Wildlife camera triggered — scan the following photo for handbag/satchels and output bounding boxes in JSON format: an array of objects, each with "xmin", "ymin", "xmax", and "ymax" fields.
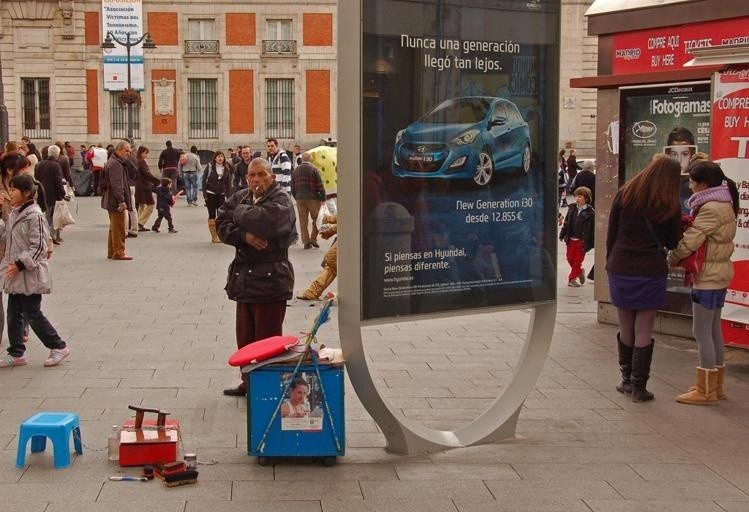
[
  {"xmin": 178, "ymin": 155, "xmax": 188, "ymax": 165},
  {"xmin": 52, "ymin": 201, "xmax": 76, "ymax": 231}
]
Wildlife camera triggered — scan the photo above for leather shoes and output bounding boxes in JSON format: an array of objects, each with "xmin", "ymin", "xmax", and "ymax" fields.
[
  {"xmin": 108, "ymin": 255, "xmax": 132, "ymax": 260},
  {"xmin": 297, "ymin": 296, "xmax": 310, "ymax": 300}
]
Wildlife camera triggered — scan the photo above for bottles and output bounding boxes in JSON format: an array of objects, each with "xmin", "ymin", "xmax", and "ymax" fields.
[
  {"xmin": 184, "ymin": 453, "xmax": 198, "ymax": 471},
  {"xmin": 107, "ymin": 425, "xmax": 121, "ymax": 461}
]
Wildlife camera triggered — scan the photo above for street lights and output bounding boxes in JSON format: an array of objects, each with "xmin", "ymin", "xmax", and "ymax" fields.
[{"xmin": 100, "ymin": 29, "xmax": 159, "ymax": 148}]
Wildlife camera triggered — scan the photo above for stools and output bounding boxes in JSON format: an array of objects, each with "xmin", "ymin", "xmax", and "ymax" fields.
[{"xmin": 15, "ymin": 412, "xmax": 83, "ymax": 468}]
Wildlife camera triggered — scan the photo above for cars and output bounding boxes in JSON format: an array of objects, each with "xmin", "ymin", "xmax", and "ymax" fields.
[
  {"xmin": 560, "ymin": 158, "xmax": 596, "ymax": 186},
  {"xmin": 389, "ymin": 93, "xmax": 531, "ymax": 190}
]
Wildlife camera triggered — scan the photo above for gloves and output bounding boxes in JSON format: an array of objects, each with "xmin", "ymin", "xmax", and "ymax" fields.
[
  {"xmin": 319, "ymin": 229, "xmax": 334, "ymax": 240},
  {"xmin": 63, "ymin": 194, "xmax": 70, "ymax": 201}
]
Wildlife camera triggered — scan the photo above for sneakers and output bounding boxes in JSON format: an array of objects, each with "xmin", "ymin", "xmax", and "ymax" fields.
[
  {"xmin": 304, "ymin": 242, "xmax": 319, "ymax": 249},
  {"xmin": 53, "ymin": 236, "xmax": 63, "ymax": 245},
  {"xmin": 187, "ymin": 200, "xmax": 198, "ymax": 206},
  {"xmin": 127, "ymin": 224, "xmax": 177, "ymax": 238},
  {"xmin": 44, "ymin": 347, "xmax": 70, "ymax": 366},
  {"xmin": 0, "ymin": 352, "xmax": 27, "ymax": 368}
]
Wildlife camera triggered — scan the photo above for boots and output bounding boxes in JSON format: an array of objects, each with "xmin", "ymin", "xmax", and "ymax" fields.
[{"xmin": 208, "ymin": 218, "xmax": 221, "ymax": 243}]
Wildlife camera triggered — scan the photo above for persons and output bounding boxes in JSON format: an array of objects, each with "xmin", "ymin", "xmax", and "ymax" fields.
[
  {"xmin": 281, "ymin": 380, "xmax": 310, "ymax": 420},
  {"xmin": 566, "ymin": 149, "xmax": 583, "ymax": 195},
  {"xmin": 568, "ymin": 159, "xmax": 596, "ymax": 210},
  {"xmin": 1, "ymin": 152, "xmax": 70, "ymax": 367},
  {"xmin": 604, "ymin": 152, "xmax": 684, "ymax": 403},
  {"xmin": 559, "ymin": 186, "xmax": 596, "ymax": 288},
  {"xmin": 0, "ymin": 136, "xmax": 337, "ymax": 259},
  {"xmin": 303, "ymin": 241, "xmax": 337, "ymax": 300},
  {"xmin": 560, "ymin": 150, "xmax": 568, "ymax": 183},
  {"xmin": 666, "ymin": 161, "xmax": 739, "ymax": 407},
  {"xmin": 215, "ymin": 157, "xmax": 297, "ymax": 396},
  {"xmin": 667, "ymin": 127, "xmax": 708, "ymax": 213}
]
